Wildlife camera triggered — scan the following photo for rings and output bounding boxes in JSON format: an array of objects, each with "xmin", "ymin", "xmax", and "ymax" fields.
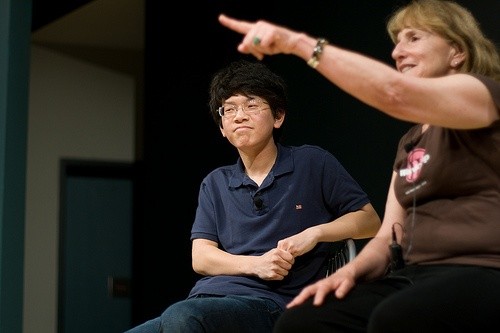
[{"xmin": 253, "ymin": 38, "xmax": 261, "ymax": 45}]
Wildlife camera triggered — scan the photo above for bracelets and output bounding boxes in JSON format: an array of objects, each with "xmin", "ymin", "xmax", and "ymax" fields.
[{"xmin": 307, "ymin": 38, "xmax": 327, "ymax": 70}]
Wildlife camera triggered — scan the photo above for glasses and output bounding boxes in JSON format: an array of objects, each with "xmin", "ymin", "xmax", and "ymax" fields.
[{"xmin": 217, "ymin": 99, "xmax": 271, "ymax": 118}]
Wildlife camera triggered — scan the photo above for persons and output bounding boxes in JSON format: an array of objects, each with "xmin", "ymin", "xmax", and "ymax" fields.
[
  {"xmin": 219, "ymin": 0, "xmax": 500, "ymax": 333},
  {"xmin": 126, "ymin": 64, "xmax": 380, "ymax": 333}
]
